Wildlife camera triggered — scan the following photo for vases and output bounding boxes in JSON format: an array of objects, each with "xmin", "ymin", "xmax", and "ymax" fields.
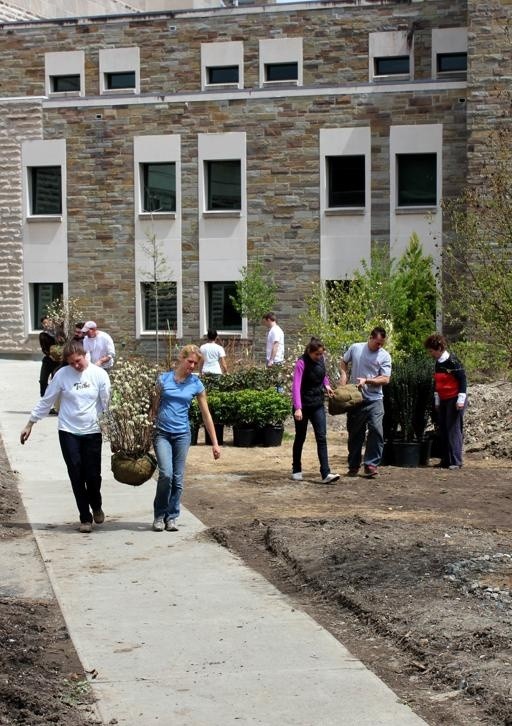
[
  {"xmin": 111, "ymin": 453, "xmax": 156, "ymax": 485},
  {"xmin": 329, "ymin": 384, "xmax": 362, "ymax": 414},
  {"xmin": 50, "ymin": 345, "xmax": 63, "ymax": 362}
]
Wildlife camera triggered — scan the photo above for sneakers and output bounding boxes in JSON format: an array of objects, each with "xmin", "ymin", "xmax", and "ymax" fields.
[
  {"xmin": 434, "ymin": 463, "xmax": 446, "ymax": 467},
  {"xmin": 348, "ymin": 467, "xmax": 358, "ymax": 476},
  {"xmin": 165, "ymin": 519, "xmax": 178, "ymax": 530},
  {"xmin": 322, "ymin": 474, "xmax": 340, "ymax": 483},
  {"xmin": 448, "ymin": 465, "xmax": 461, "ymax": 469},
  {"xmin": 154, "ymin": 514, "xmax": 165, "ymax": 531},
  {"xmin": 93, "ymin": 509, "xmax": 104, "ymax": 523},
  {"xmin": 80, "ymin": 523, "xmax": 92, "ymax": 531},
  {"xmin": 293, "ymin": 472, "xmax": 302, "ymax": 480},
  {"xmin": 364, "ymin": 465, "xmax": 379, "ymax": 476}
]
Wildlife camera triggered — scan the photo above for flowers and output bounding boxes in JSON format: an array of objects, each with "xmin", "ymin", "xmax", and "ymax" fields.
[
  {"xmin": 47, "ymin": 298, "xmax": 85, "ymax": 345},
  {"xmin": 97, "ymin": 355, "xmax": 157, "ymax": 456},
  {"xmin": 278, "ymin": 340, "xmax": 341, "ymax": 387}
]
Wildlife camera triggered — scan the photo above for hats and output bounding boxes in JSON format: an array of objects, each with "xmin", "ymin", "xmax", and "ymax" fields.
[{"xmin": 81, "ymin": 321, "xmax": 97, "ymax": 333}]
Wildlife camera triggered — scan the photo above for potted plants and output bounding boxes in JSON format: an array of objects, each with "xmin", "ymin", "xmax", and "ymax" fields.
[
  {"xmin": 188, "ymin": 396, "xmax": 199, "ymax": 445},
  {"xmin": 382, "ymin": 353, "xmax": 432, "ymax": 466},
  {"xmin": 199, "ymin": 391, "xmax": 226, "ymax": 446},
  {"xmin": 226, "ymin": 389, "xmax": 291, "ymax": 446}
]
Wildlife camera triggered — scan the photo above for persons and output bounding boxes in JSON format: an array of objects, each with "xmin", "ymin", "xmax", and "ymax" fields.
[
  {"xmin": 151, "ymin": 344, "xmax": 221, "ymax": 532},
  {"xmin": 48, "ymin": 322, "xmax": 85, "ymax": 415},
  {"xmin": 19, "ymin": 340, "xmax": 112, "ymax": 533},
  {"xmin": 80, "ymin": 320, "xmax": 115, "ymax": 372},
  {"xmin": 261, "ymin": 312, "xmax": 285, "ymax": 392},
  {"xmin": 198, "ymin": 327, "xmax": 228, "ymax": 378},
  {"xmin": 423, "ymin": 333, "xmax": 470, "ymax": 471},
  {"xmin": 39, "ymin": 316, "xmax": 66, "ymax": 397},
  {"xmin": 338, "ymin": 325, "xmax": 392, "ymax": 478},
  {"xmin": 290, "ymin": 335, "xmax": 341, "ymax": 484}
]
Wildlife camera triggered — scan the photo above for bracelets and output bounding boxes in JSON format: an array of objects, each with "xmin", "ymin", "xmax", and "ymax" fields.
[{"xmin": 365, "ymin": 377, "xmax": 367, "ymax": 384}]
